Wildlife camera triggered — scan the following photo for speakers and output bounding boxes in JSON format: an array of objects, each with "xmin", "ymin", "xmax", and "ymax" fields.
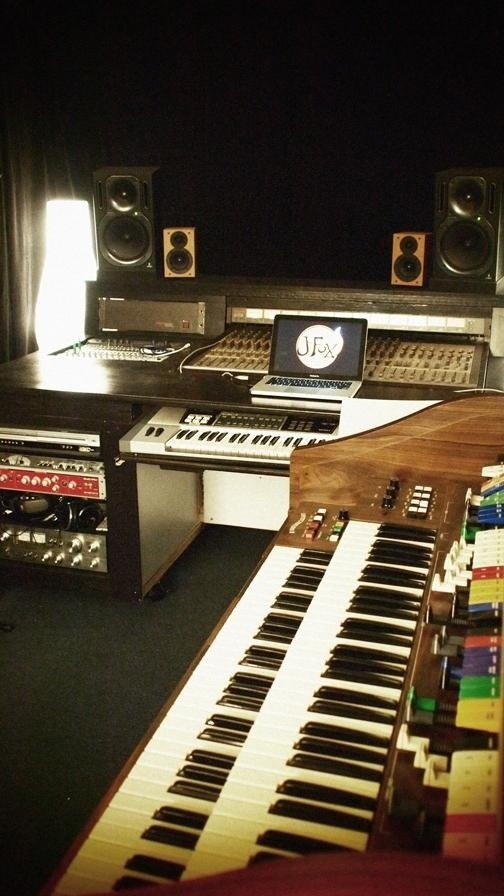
[
  {"xmin": 90, "ymin": 162, "xmax": 159, "ymax": 282},
  {"xmin": 390, "ymin": 231, "xmax": 433, "ymax": 287},
  {"xmin": 428, "ymin": 165, "xmax": 504, "ymax": 296},
  {"xmin": 162, "ymin": 226, "xmax": 196, "ymax": 279}
]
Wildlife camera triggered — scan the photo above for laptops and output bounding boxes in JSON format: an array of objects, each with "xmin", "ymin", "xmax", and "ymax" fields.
[{"xmin": 248, "ymin": 313, "xmax": 369, "ymax": 404}]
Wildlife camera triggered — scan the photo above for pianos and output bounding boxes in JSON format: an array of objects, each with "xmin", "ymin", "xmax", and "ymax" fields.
[
  {"xmin": 118, "ymin": 406, "xmax": 339, "ymax": 469},
  {"xmin": 53, "ymin": 393, "xmax": 502, "ymax": 896}
]
